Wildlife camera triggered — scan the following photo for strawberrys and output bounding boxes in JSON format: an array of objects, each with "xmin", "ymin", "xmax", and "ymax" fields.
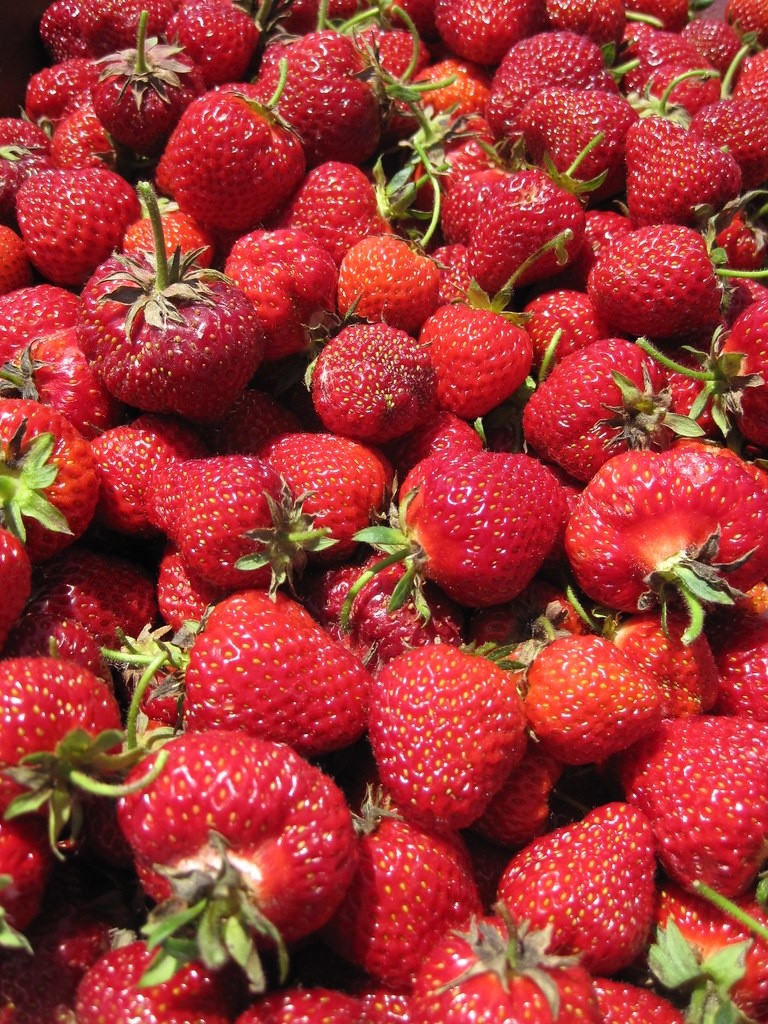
[{"xmin": 0, "ymin": 0, "xmax": 768, "ymax": 1024}]
